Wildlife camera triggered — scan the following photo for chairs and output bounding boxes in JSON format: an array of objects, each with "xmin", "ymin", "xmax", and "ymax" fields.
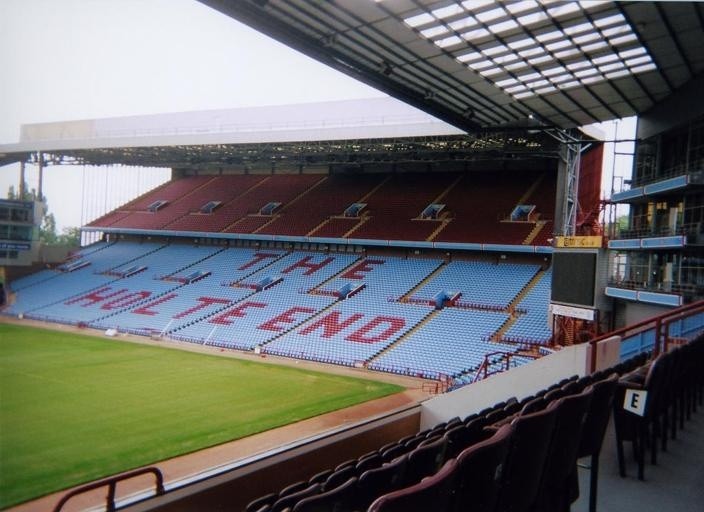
[
  {"xmin": 232, "ymin": 245, "xmax": 553, "ymax": 350},
  {"xmin": 83, "ymin": 175, "xmax": 557, "ymax": 247},
  {"xmin": 1, "ymin": 239, "xmax": 232, "ymax": 350},
  {"xmin": 242, "ymin": 336, "xmax": 703, "ymax": 512}
]
